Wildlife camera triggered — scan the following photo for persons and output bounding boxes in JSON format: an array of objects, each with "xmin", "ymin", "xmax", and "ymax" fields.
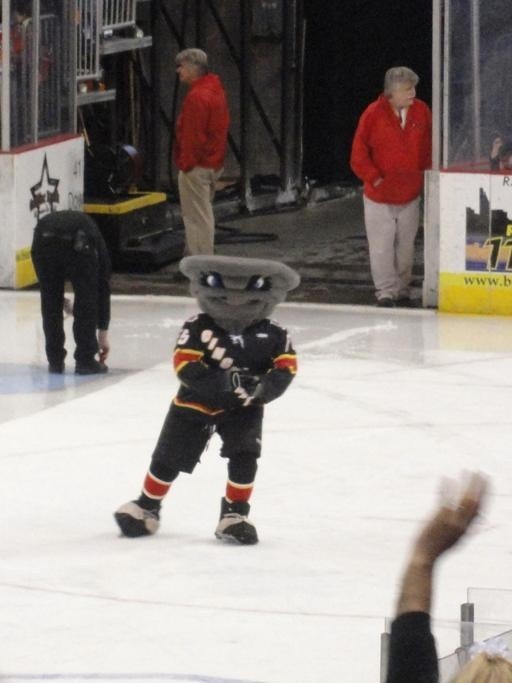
[
  {"xmin": 1, "ymin": 0, "xmax": 44, "ymax": 145},
  {"xmin": 28, "ymin": 210, "xmax": 115, "ymax": 372},
  {"xmin": 384, "ymin": 467, "xmax": 511, "ymax": 681},
  {"xmin": 174, "ymin": 47, "xmax": 233, "ymax": 258},
  {"xmin": 349, "ymin": 65, "xmax": 434, "ymax": 311},
  {"xmin": 488, "ymin": 137, "xmax": 512, "ymax": 170}
]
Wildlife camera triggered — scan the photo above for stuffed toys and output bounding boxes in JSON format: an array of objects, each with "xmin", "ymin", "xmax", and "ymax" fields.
[{"xmin": 116, "ymin": 253, "xmax": 302, "ymax": 546}]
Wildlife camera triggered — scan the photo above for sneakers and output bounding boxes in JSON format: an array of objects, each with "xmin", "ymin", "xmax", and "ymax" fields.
[
  {"xmin": 378, "ymin": 297, "xmax": 391, "ymax": 308},
  {"xmin": 47, "ymin": 363, "xmax": 66, "ymax": 373},
  {"xmin": 397, "ymin": 297, "xmax": 411, "ymax": 308},
  {"xmin": 76, "ymin": 361, "xmax": 109, "ymax": 374}
]
